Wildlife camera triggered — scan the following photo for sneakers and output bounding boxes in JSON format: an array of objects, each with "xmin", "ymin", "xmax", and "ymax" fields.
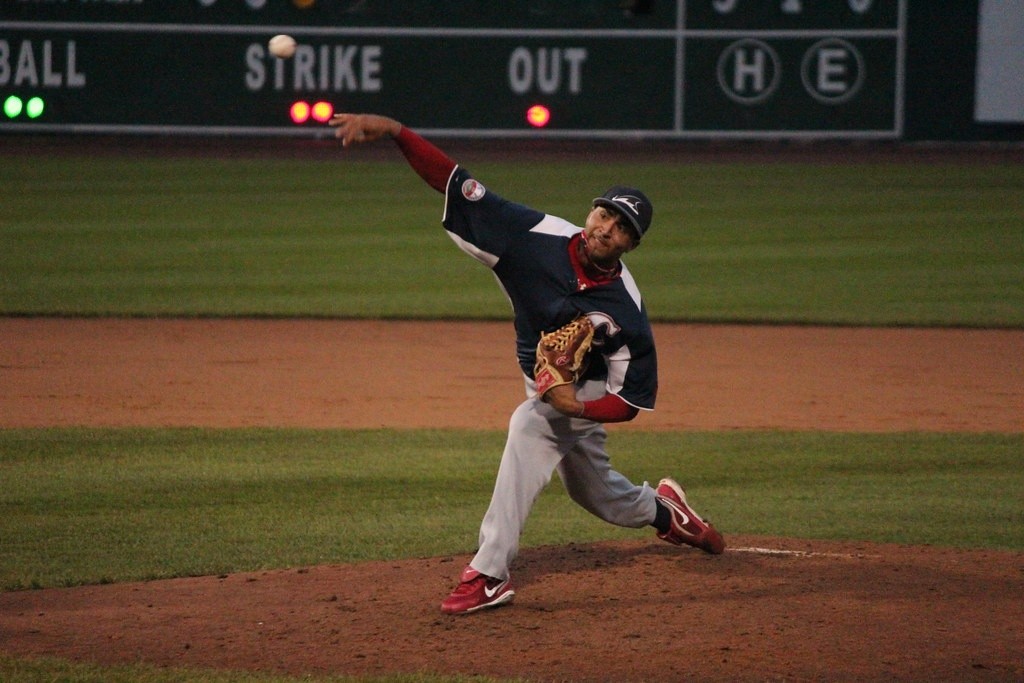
[
  {"xmin": 655, "ymin": 477, "xmax": 726, "ymax": 553},
  {"xmin": 442, "ymin": 567, "xmax": 516, "ymax": 615}
]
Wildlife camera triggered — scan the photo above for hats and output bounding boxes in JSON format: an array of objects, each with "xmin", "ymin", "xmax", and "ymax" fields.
[{"xmin": 593, "ymin": 185, "xmax": 654, "ymax": 238}]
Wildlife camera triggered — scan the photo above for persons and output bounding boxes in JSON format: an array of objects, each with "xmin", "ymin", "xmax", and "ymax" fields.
[{"xmin": 327, "ymin": 111, "xmax": 726, "ymax": 615}]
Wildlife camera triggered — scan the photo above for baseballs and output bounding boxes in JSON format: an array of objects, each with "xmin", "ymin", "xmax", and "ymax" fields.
[{"xmin": 268, "ymin": 34, "xmax": 296, "ymax": 58}]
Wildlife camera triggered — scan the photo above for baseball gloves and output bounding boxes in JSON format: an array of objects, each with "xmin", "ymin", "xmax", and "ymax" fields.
[{"xmin": 533, "ymin": 315, "xmax": 594, "ymax": 403}]
